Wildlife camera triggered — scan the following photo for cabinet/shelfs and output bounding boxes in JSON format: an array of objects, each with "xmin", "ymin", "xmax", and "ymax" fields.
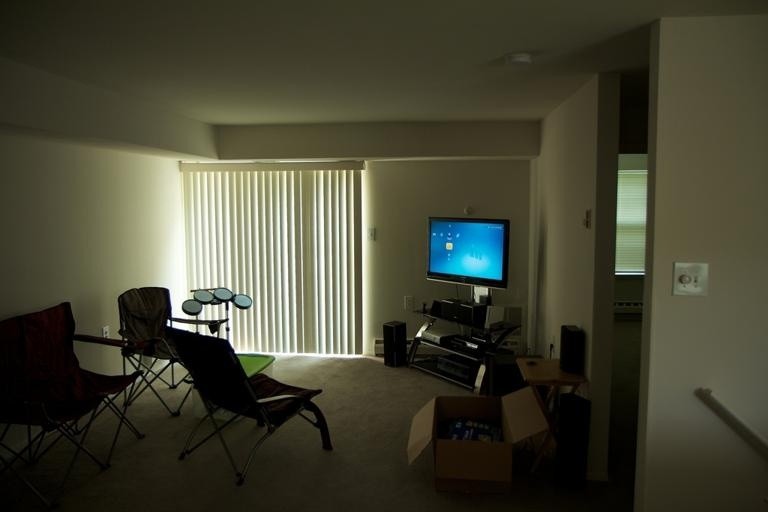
[{"xmin": 406, "ymin": 309, "xmax": 523, "ymax": 397}]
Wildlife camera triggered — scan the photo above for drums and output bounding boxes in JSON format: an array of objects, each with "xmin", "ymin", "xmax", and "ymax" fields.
[
  {"xmin": 214, "ymin": 289, "xmax": 233, "ymax": 300},
  {"xmin": 182, "ymin": 299, "xmax": 202, "ymax": 315},
  {"xmin": 233, "ymin": 294, "xmax": 252, "ymax": 309},
  {"xmin": 194, "ymin": 290, "xmax": 213, "ymax": 304}
]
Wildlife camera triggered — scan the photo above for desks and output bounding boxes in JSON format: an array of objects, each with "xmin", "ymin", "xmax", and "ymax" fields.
[{"xmin": 514, "ymin": 357, "xmax": 586, "ymax": 488}]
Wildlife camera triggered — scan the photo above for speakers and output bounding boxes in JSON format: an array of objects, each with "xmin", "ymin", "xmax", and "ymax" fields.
[
  {"xmin": 382, "ymin": 320, "xmax": 408, "ymax": 368},
  {"xmin": 559, "ymin": 324, "xmax": 586, "ymax": 375}
]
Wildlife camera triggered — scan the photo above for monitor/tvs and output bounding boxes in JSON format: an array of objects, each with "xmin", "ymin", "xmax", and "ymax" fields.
[{"xmin": 425, "ymin": 216, "xmax": 510, "ymax": 292}]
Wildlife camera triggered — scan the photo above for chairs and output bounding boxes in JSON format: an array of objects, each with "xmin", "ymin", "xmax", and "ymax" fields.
[
  {"xmin": 116, "ymin": 285, "xmax": 213, "ymax": 418},
  {"xmin": 1, "ymin": 300, "xmax": 145, "ymax": 509},
  {"xmin": 163, "ymin": 325, "xmax": 333, "ymax": 487}
]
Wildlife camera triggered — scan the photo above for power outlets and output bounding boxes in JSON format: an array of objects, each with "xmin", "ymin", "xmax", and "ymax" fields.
[{"xmin": 102, "ymin": 324, "xmax": 110, "ymax": 339}]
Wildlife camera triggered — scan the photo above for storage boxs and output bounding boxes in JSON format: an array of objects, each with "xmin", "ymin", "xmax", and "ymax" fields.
[
  {"xmin": 406, "ymin": 385, "xmax": 550, "ymax": 498},
  {"xmin": 184, "ymin": 353, "xmax": 275, "ymax": 424}
]
